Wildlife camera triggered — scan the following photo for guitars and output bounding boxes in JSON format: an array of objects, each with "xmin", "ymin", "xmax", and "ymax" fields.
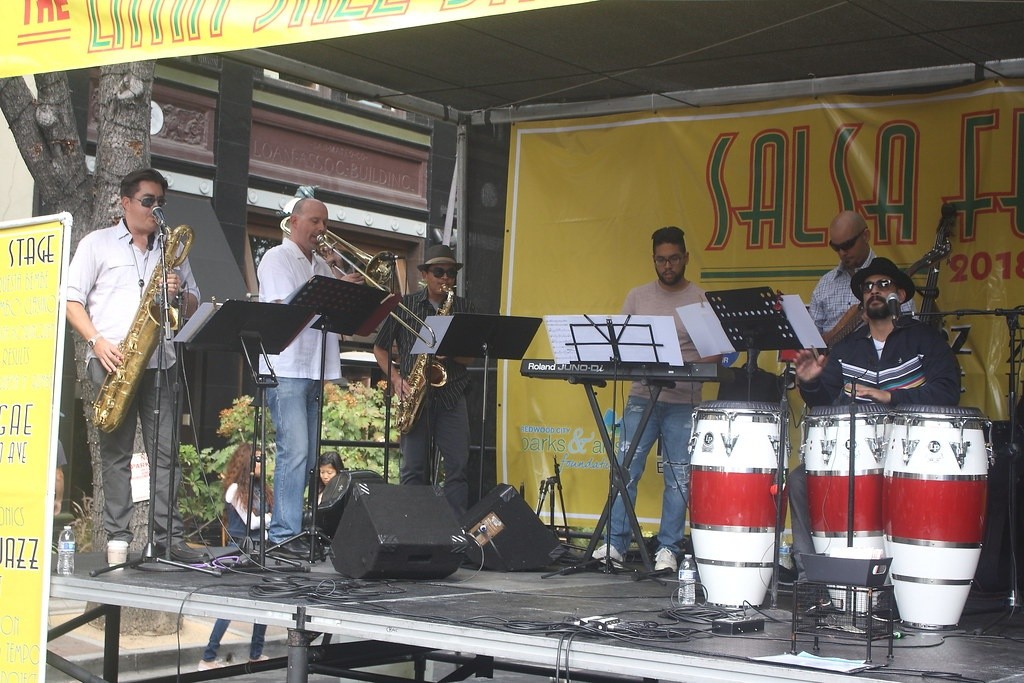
[
  {"xmin": 795, "ymin": 238, "xmax": 953, "ymax": 385},
  {"xmin": 916, "ymin": 201, "xmax": 959, "ymax": 325}
]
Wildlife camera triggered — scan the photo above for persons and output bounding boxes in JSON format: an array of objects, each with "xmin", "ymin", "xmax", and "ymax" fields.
[
  {"xmin": 197, "ymin": 442, "xmax": 274, "ymax": 671},
  {"xmin": 54, "ymin": 438, "xmax": 68, "ymax": 518},
  {"xmin": 257, "ymin": 197, "xmax": 379, "ymax": 556},
  {"xmin": 593, "ymin": 228, "xmax": 721, "ymax": 571},
  {"xmin": 307, "ymin": 450, "xmax": 344, "ymax": 510},
  {"xmin": 59, "ymin": 169, "xmax": 210, "ymax": 563},
  {"xmin": 805, "ymin": 210, "xmax": 919, "ymax": 406},
  {"xmin": 793, "ymin": 256, "xmax": 961, "ymax": 593},
  {"xmin": 374, "ymin": 245, "xmax": 474, "ymax": 526}
]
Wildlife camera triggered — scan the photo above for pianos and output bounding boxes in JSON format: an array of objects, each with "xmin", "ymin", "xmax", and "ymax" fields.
[{"xmin": 519, "ymin": 359, "xmax": 730, "ymax": 582}]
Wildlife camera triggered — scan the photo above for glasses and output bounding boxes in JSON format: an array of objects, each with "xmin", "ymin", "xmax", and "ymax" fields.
[
  {"xmin": 653, "ymin": 254, "xmax": 687, "ymax": 267},
  {"xmin": 828, "ymin": 227, "xmax": 868, "ymax": 253},
  {"xmin": 859, "ymin": 278, "xmax": 899, "ymax": 293},
  {"xmin": 129, "ymin": 195, "xmax": 167, "ymax": 208},
  {"xmin": 424, "ymin": 267, "xmax": 458, "ymax": 279}
]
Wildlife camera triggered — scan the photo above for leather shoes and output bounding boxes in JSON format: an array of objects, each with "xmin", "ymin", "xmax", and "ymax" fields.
[
  {"xmin": 156, "ymin": 541, "xmax": 210, "ymax": 564},
  {"xmin": 266, "ymin": 538, "xmax": 311, "ymax": 559},
  {"xmin": 105, "ymin": 544, "xmax": 130, "ymax": 563}
]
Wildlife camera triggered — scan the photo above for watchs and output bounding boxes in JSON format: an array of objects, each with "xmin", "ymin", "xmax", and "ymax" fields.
[
  {"xmin": 171, "ymin": 293, "xmax": 183, "ymax": 308},
  {"xmin": 87, "ymin": 334, "xmax": 100, "ymax": 348}
]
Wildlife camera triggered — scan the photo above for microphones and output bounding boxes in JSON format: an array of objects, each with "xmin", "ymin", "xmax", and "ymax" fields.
[
  {"xmin": 886, "ymin": 293, "xmax": 900, "ymax": 316},
  {"xmin": 380, "ymin": 252, "xmax": 404, "ymax": 259},
  {"xmin": 152, "ymin": 206, "xmax": 166, "ymax": 228}
]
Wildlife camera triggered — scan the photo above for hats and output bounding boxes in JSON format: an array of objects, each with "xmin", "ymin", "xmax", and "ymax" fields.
[
  {"xmin": 850, "ymin": 257, "xmax": 915, "ymax": 305},
  {"xmin": 417, "ymin": 245, "xmax": 464, "ymax": 272}
]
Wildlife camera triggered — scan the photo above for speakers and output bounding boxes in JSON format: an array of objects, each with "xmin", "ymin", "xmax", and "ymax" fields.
[
  {"xmin": 460, "ymin": 485, "xmax": 562, "ymax": 571},
  {"xmin": 327, "ymin": 483, "xmax": 467, "ymax": 579}
]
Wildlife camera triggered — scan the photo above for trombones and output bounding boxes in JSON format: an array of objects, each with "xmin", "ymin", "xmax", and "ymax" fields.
[{"xmin": 280, "ymin": 215, "xmax": 436, "ymax": 349}]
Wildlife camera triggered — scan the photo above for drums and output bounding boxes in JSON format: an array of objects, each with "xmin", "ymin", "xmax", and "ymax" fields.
[
  {"xmin": 801, "ymin": 404, "xmax": 891, "ymax": 617},
  {"xmin": 882, "ymin": 402, "xmax": 989, "ymax": 635},
  {"xmin": 688, "ymin": 401, "xmax": 790, "ymax": 609}
]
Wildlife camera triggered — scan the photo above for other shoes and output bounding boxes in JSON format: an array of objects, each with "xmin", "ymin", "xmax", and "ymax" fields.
[
  {"xmin": 258, "ymin": 654, "xmax": 269, "ymax": 661},
  {"xmin": 198, "ymin": 660, "xmax": 224, "ymax": 671}
]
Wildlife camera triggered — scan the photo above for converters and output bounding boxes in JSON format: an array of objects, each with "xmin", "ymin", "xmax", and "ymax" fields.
[{"xmin": 711, "ymin": 618, "xmax": 764, "ymax": 636}]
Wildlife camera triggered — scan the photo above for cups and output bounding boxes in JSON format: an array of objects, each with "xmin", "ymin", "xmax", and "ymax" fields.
[{"xmin": 108, "ymin": 540, "xmax": 129, "ymax": 571}]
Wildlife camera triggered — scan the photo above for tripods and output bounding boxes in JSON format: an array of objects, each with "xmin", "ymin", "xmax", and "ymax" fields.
[
  {"xmin": 539, "ymin": 314, "xmax": 666, "ymax": 589},
  {"xmin": 88, "ymin": 228, "xmax": 389, "ymax": 578},
  {"xmin": 894, "ymin": 309, "xmax": 1024, "ymax": 636}
]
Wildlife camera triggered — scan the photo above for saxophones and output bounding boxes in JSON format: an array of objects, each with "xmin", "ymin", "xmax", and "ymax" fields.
[
  {"xmin": 388, "ymin": 283, "xmax": 467, "ymax": 435},
  {"xmin": 90, "ymin": 206, "xmax": 202, "ymax": 434}
]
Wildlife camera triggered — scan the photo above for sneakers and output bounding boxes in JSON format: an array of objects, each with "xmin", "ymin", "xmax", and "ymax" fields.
[
  {"xmin": 592, "ymin": 543, "xmax": 624, "ymax": 565},
  {"xmin": 654, "ymin": 547, "xmax": 677, "ymax": 573}
]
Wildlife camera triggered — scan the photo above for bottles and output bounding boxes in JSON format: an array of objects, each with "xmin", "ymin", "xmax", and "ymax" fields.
[
  {"xmin": 678, "ymin": 554, "xmax": 697, "ymax": 605},
  {"xmin": 779, "ymin": 530, "xmax": 795, "ymax": 569},
  {"xmin": 56, "ymin": 525, "xmax": 75, "ymax": 575}
]
